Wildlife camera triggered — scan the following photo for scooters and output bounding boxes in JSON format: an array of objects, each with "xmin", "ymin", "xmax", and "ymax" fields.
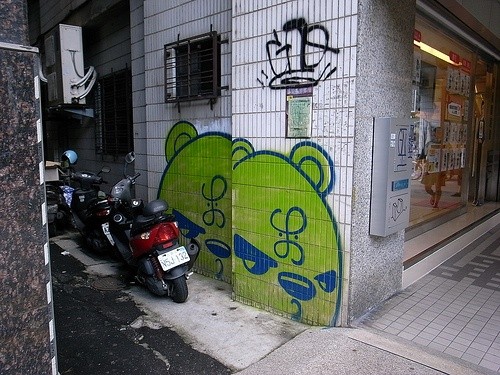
[
  {"xmin": 95, "ymin": 153, "xmax": 199, "ymax": 303},
  {"xmin": 40, "ymin": 152, "xmax": 81, "ymax": 237},
  {"xmin": 56, "ymin": 151, "xmax": 119, "ymax": 257}
]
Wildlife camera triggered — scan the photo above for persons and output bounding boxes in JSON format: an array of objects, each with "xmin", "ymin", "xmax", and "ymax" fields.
[{"xmin": 414, "ymin": 126, "xmax": 456, "ymax": 208}]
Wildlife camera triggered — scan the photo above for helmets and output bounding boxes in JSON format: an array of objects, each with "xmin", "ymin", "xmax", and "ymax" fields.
[{"xmin": 60, "ymin": 150, "xmax": 78, "ymax": 164}]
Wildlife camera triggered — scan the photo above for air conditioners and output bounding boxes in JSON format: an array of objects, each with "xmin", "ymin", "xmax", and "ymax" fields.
[{"xmin": 42, "ymin": 24, "xmax": 86, "ymax": 108}]
